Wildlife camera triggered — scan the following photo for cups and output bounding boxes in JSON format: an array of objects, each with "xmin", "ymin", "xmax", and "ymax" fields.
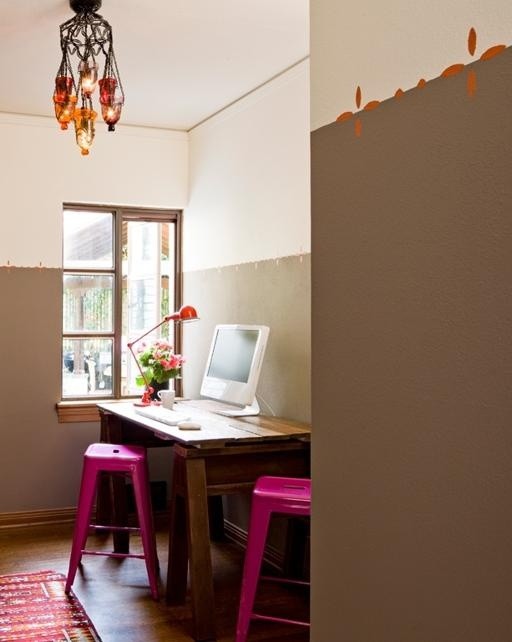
[{"xmin": 157, "ymin": 390, "xmax": 176, "ymax": 410}]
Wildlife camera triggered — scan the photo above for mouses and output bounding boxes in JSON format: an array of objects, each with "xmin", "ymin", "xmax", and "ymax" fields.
[{"xmin": 176, "ymin": 421, "xmax": 202, "ymax": 431}]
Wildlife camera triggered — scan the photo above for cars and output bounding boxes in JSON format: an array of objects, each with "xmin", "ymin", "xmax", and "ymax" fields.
[{"xmin": 64, "ymin": 351, "xmax": 90, "ymax": 373}]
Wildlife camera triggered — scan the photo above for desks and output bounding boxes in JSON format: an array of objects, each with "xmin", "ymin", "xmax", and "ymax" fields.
[{"xmin": 96, "ymin": 398, "xmax": 311, "ymax": 642}]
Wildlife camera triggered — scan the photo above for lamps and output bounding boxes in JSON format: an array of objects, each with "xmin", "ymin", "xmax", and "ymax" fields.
[
  {"xmin": 127, "ymin": 305, "xmax": 200, "ymax": 407},
  {"xmin": 52, "ymin": 0, "xmax": 124, "ymax": 155}
]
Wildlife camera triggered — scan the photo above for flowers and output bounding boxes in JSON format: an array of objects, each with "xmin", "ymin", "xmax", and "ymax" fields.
[{"xmin": 137, "ymin": 341, "xmax": 182, "ymax": 382}]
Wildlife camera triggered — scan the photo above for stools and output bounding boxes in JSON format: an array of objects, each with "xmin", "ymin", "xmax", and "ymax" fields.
[
  {"xmin": 64, "ymin": 442, "xmax": 161, "ymax": 601},
  {"xmin": 235, "ymin": 475, "xmax": 312, "ymax": 642}
]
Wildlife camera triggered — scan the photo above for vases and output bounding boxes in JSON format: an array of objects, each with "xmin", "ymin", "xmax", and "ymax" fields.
[{"xmin": 146, "ymin": 379, "xmax": 170, "ymax": 397}]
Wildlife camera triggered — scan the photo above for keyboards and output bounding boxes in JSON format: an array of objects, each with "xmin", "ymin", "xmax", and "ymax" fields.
[{"xmin": 136, "ymin": 405, "xmax": 191, "ymax": 425}]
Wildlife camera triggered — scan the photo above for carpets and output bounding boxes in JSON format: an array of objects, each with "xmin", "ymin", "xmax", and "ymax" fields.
[{"xmin": 0, "ymin": 571, "xmax": 100, "ymax": 642}]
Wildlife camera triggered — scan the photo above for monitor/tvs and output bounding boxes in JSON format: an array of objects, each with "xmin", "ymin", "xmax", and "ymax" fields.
[{"xmin": 200, "ymin": 323, "xmax": 270, "ymax": 417}]
